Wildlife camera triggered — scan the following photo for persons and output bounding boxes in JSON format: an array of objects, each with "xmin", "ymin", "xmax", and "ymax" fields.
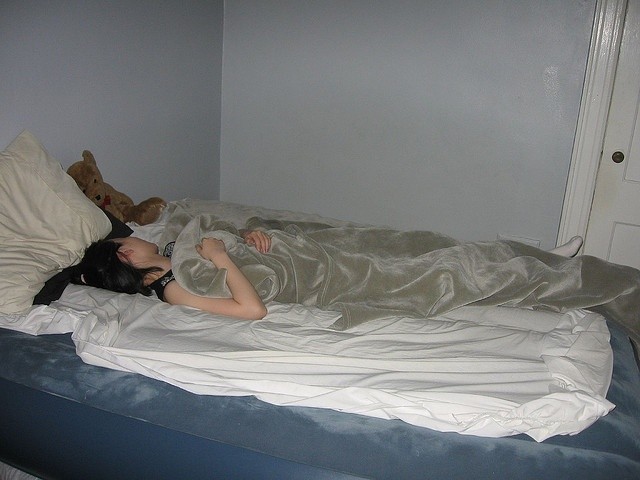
[{"xmin": 32, "ymin": 231, "xmax": 583, "ymax": 320}]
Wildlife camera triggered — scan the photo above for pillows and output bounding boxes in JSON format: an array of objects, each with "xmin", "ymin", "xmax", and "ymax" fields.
[{"xmin": 0, "ymin": 128, "xmax": 113, "ymax": 322}]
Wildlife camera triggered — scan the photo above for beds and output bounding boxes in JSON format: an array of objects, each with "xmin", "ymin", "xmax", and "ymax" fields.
[{"xmin": 0, "ymin": 193, "xmax": 639, "ymax": 480}]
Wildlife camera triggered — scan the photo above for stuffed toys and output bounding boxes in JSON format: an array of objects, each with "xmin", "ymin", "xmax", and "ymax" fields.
[{"xmin": 67, "ymin": 150, "xmax": 166, "ymax": 226}]
